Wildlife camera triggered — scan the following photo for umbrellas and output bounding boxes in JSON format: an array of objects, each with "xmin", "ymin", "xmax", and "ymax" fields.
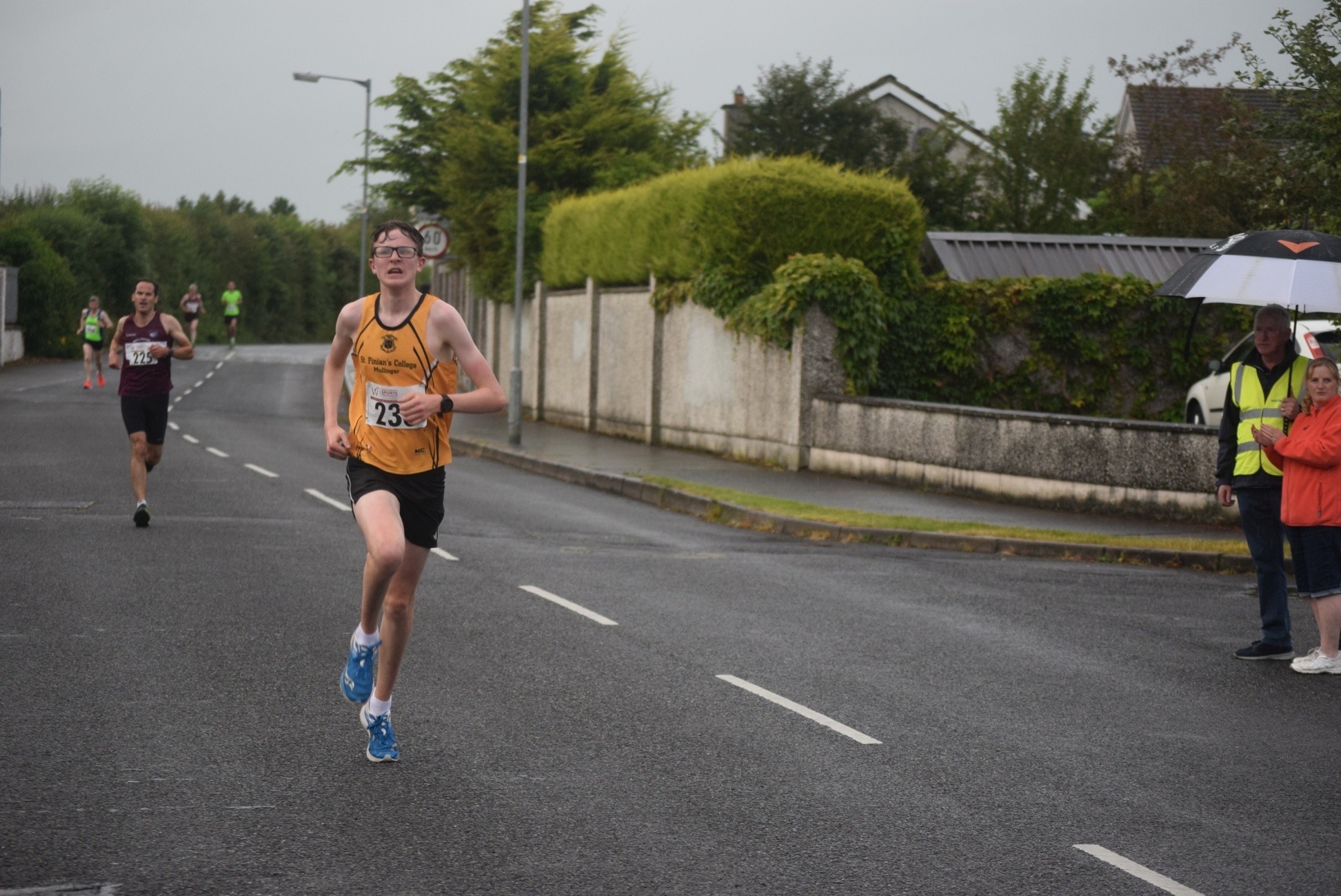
[{"xmin": 1147, "ymin": 230, "xmax": 1341, "ymax": 438}]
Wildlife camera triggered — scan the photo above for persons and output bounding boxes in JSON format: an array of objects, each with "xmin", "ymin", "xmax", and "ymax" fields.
[
  {"xmin": 77, "ymin": 295, "xmax": 113, "ymax": 390},
  {"xmin": 220, "ymin": 279, "xmax": 241, "ymax": 351},
  {"xmin": 109, "ymin": 278, "xmax": 194, "ymax": 526},
  {"xmin": 1213, "ymin": 304, "xmax": 1315, "ymax": 660},
  {"xmin": 181, "ymin": 284, "xmax": 207, "ymax": 345},
  {"xmin": 1249, "ymin": 355, "xmax": 1341, "ymax": 676},
  {"xmin": 323, "ymin": 220, "xmax": 512, "ymax": 763}
]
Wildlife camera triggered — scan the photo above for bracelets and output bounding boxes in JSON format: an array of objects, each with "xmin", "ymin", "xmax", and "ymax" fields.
[{"xmin": 166, "ymin": 346, "xmax": 174, "ymax": 358}]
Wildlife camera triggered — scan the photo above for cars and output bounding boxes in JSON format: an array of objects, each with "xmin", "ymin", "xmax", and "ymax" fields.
[{"xmin": 1183, "ymin": 319, "xmax": 1341, "ymax": 427}]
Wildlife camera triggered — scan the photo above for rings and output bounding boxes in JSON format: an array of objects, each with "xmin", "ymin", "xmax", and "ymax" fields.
[{"xmin": 1281, "ymin": 409, "xmax": 1285, "ymax": 412}]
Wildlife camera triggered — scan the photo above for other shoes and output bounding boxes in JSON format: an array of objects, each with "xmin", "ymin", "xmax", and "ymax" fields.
[
  {"xmin": 83, "ymin": 379, "xmax": 93, "ymax": 389},
  {"xmin": 96, "ymin": 371, "xmax": 105, "ymax": 386}
]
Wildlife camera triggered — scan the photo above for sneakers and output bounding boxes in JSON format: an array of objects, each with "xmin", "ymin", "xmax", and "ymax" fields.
[
  {"xmin": 145, "ymin": 459, "xmax": 154, "ymax": 473},
  {"xmin": 339, "ymin": 629, "xmax": 382, "ymax": 706},
  {"xmin": 1233, "ymin": 639, "xmax": 1295, "ymax": 661},
  {"xmin": 133, "ymin": 503, "xmax": 151, "ymax": 528},
  {"xmin": 1290, "ymin": 646, "xmax": 1341, "ymax": 675},
  {"xmin": 359, "ymin": 700, "xmax": 401, "ymax": 763}
]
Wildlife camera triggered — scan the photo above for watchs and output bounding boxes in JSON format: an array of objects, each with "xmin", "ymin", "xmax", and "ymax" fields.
[{"xmin": 437, "ymin": 394, "xmax": 453, "ymax": 418}]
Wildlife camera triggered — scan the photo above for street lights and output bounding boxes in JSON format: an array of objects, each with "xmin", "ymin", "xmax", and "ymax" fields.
[{"xmin": 293, "ymin": 70, "xmax": 372, "ymax": 298}]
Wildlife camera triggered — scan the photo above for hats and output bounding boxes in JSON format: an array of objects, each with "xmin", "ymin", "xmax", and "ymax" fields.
[{"xmin": 90, "ymin": 296, "xmax": 99, "ymax": 302}]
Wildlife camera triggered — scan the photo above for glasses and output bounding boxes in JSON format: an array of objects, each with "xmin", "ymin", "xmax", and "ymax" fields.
[{"xmin": 372, "ymin": 246, "xmax": 422, "ymax": 259}]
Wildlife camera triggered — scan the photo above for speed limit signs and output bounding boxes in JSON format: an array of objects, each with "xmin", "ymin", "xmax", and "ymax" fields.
[{"xmin": 417, "ymin": 223, "xmax": 450, "ymax": 259}]
